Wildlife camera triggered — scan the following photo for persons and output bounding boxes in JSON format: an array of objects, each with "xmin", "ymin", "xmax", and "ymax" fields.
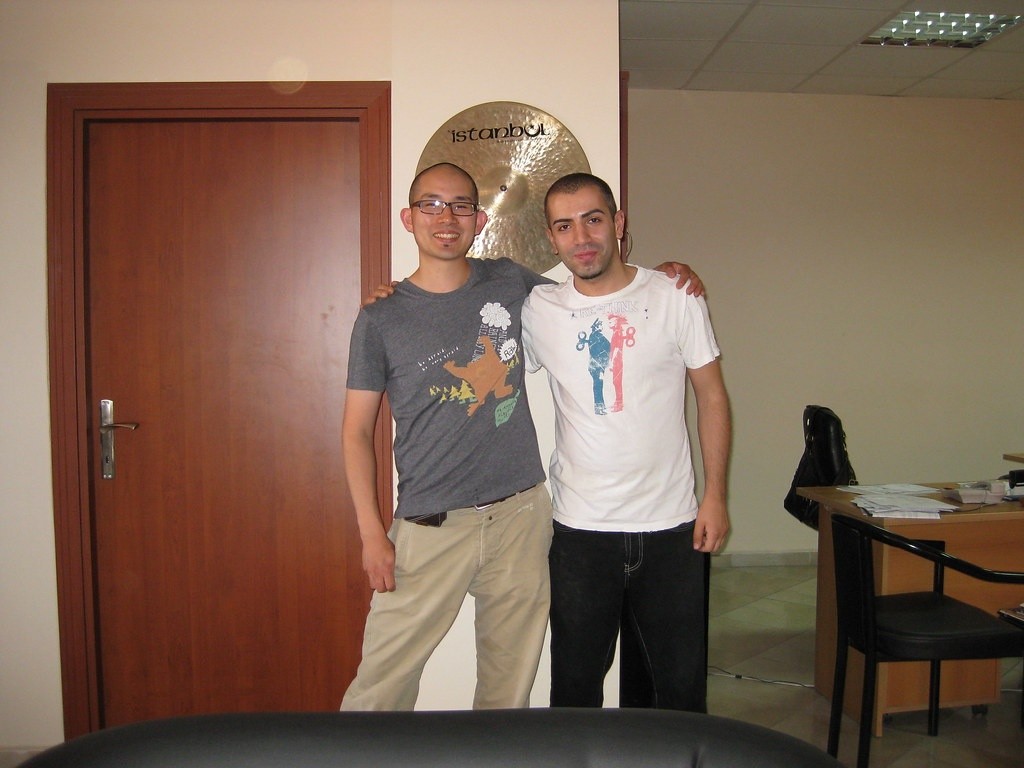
[
  {"xmin": 358, "ymin": 174, "xmax": 733, "ymax": 712},
  {"xmin": 341, "ymin": 163, "xmax": 705, "ymax": 710}
]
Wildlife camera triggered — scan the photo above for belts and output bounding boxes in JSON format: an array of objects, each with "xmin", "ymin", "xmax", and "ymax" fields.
[{"xmin": 464, "ymin": 485, "xmax": 535, "ymax": 510}]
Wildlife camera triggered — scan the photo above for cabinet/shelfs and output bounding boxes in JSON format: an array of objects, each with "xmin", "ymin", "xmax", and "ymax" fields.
[{"xmin": 795, "ymin": 479, "xmax": 1024, "ymax": 739}]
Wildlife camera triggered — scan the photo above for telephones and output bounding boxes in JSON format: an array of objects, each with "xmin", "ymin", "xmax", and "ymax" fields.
[{"xmin": 942, "ymin": 479, "xmax": 1006, "ymax": 504}]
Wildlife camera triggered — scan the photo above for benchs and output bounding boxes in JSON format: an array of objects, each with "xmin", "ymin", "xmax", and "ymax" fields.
[{"xmin": 15, "ymin": 708, "xmax": 842, "ymax": 768}]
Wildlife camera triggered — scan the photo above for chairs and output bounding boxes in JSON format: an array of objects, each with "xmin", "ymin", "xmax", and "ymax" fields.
[
  {"xmin": 784, "ymin": 405, "xmax": 860, "ymax": 532},
  {"xmin": 827, "ymin": 512, "xmax": 1024, "ymax": 768}
]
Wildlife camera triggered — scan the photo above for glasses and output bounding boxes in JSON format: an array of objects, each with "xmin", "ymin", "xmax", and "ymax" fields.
[{"xmin": 410, "ymin": 200, "xmax": 480, "ymax": 216}]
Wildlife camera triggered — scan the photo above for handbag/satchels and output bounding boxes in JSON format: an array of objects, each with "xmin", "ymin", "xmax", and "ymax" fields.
[{"xmin": 783, "ymin": 406, "xmax": 846, "ymax": 531}]
